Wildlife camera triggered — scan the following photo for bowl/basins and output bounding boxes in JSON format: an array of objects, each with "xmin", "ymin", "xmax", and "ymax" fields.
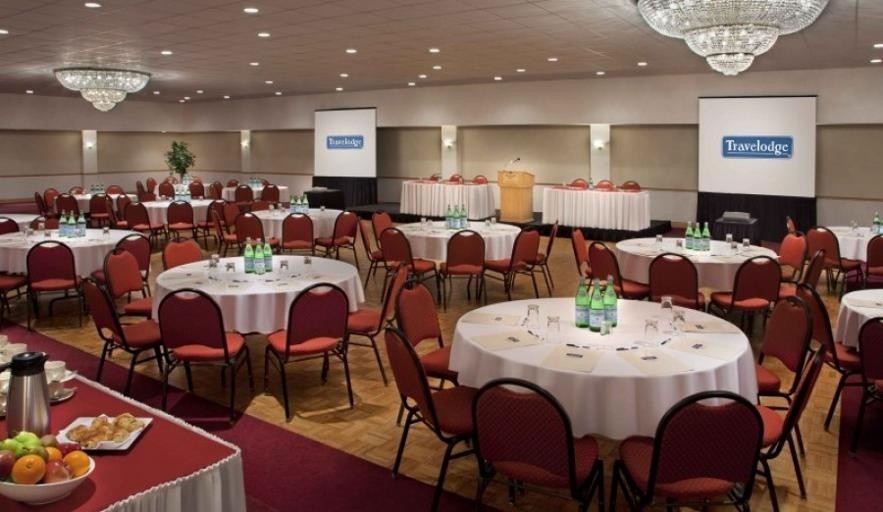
[
  {"xmin": 0, "ymin": 448, "xmax": 95, "ymax": 504},
  {"xmin": 0, "ymin": 334, "xmax": 27, "ymax": 364},
  {"xmin": 43, "ymin": 359, "xmax": 66, "ymax": 379}
]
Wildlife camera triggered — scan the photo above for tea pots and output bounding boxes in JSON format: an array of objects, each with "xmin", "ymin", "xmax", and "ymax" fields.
[{"xmin": 0, "ymin": 351, "xmax": 52, "ymax": 439}]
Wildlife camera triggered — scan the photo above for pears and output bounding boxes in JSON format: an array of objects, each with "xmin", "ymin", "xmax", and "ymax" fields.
[{"xmin": 0, "ymin": 431, "xmax": 45, "ymax": 460}]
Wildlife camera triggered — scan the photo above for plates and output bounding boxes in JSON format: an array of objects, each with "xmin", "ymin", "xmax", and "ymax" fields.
[
  {"xmin": 54, "ymin": 415, "xmax": 153, "ymax": 451},
  {"xmin": 45, "ymin": 369, "xmax": 77, "ymax": 404}
]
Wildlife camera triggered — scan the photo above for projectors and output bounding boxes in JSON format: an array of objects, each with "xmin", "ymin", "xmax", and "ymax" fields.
[
  {"xmin": 311, "ymin": 186, "xmax": 328, "ymax": 192},
  {"xmin": 725, "ymin": 210, "xmax": 751, "ymax": 223}
]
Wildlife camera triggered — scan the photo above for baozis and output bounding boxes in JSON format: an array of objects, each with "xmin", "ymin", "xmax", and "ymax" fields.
[{"xmin": 65, "ymin": 412, "xmax": 145, "ymax": 449}]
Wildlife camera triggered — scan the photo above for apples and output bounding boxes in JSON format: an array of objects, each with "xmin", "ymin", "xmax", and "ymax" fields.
[
  {"xmin": 0, "ymin": 450, "xmax": 15, "ymax": 481},
  {"xmin": 44, "ymin": 459, "xmax": 73, "ymax": 483}
]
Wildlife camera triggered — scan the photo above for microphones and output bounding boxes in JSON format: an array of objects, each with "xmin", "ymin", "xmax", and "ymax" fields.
[{"xmin": 511, "ymin": 157, "xmax": 522, "ymax": 164}]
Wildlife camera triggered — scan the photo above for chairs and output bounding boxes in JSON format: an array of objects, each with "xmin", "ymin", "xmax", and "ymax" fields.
[
  {"xmin": 378, "ymin": 227, "xmax": 439, "ymax": 308},
  {"xmin": 756, "ymin": 342, "xmax": 830, "ymax": 511},
  {"xmin": 401, "ymin": 174, "xmax": 497, "ymax": 220},
  {"xmin": 864, "ymin": 233, "xmax": 882, "ymax": 288},
  {"xmin": 379, "ymin": 326, "xmax": 476, "ymax": 510},
  {"xmin": 358, "ymin": 219, "xmax": 386, "ymax": 291},
  {"xmin": 155, "ymin": 288, "xmax": 253, "ymax": 433},
  {"xmin": 532, "ymin": 219, "xmax": 559, "ymax": 297},
  {"xmin": 811, "ymin": 226, "xmax": 861, "ymax": 302},
  {"xmin": 571, "ymin": 226, "xmax": 588, "ymax": 279},
  {"xmin": 753, "ymin": 296, "xmax": 810, "ymax": 456},
  {"xmin": 24, "ymin": 240, "xmax": 84, "ymax": 329},
  {"xmin": 796, "ymin": 279, "xmax": 866, "ymax": 431},
  {"xmin": 161, "ymin": 236, "xmax": 202, "ymax": 269},
  {"xmin": 393, "ymin": 277, "xmax": 456, "ymax": 429},
  {"xmin": 616, "ymin": 389, "xmax": 766, "ymax": 512},
  {"xmin": 485, "ymin": 225, "xmax": 540, "ymax": 301},
  {"xmin": 346, "ymin": 263, "xmax": 408, "ymax": 388},
  {"xmin": 0, "ymin": 273, "xmax": 31, "ymax": 322},
  {"xmin": 780, "ymin": 249, "xmax": 824, "ymax": 301},
  {"xmin": 644, "ymin": 253, "xmax": 705, "ymax": 309},
  {"xmin": 784, "ymin": 215, "xmax": 796, "ymax": 234},
  {"xmin": 708, "ymin": 256, "xmax": 783, "ymax": 337},
  {"xmin": 587, "ymin": 241, "xmax": 644, "ymax": 301},
  {"xmin": 33, "ymin": 175, "xmax": 360, "ymax": 263},
  {"xmin": 805, "ymin": 228, "xmax": 835, "ymax": 288},
  {"xmin": 371, "ymin": 211, "xmax": 393, "ymax": 250},
  {"xmin": 465, "ymin": 376, "xmax": 606, "ymax": 512},
  {"xmin": 80, "ymin": 277, "xmax": 164, "ymax": 394},
  {"xmin": 438, "ymin": 230, "xmax": 486, "ymax": 314},
  {"xmin": 849, "ymin": 316, "xmax": 883, "ymax": 454},
  {"xmin": 264, "ymin": 280, "xmax": 354, "ymax": 420},
  {"xmin": 114, "ymin": 234, "xmax": 152, "ymax": 298},
  {"xmin": 101, "ymin": 247, "xmax": 154, "ymax": 321},
  {"xmin": 543, "ymin": 178, "xmax": 653, "ymax": 235},
  {"xmin": 778, "ymin": 230, "xmax": 807, "ymax": 282}
]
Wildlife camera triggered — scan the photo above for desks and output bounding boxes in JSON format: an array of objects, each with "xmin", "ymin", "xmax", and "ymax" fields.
[
  {"xmin": 615, "ymin": 235, "xmax": 777, "ymax": 302},
  {"xmin": 391, "ymin": 218, "xmax": 522, "ymax": 300},
  {"xmin": 840, "ymin": 286, "xmax": 882, "ymax": 354},
  {"xmin": 818, "ymin": 222, "xmax": 883, "ymax": 265},
  {"xmin": 160, "ymin": 254, "xmax": 370, "ymax": 351},
  {"xmin": 3, "ymin": 228, "xmax": 135, "ymax": 307},
  {"xmin": 439, "ymin": 295, "xmax": 759, "ymax": 491}
]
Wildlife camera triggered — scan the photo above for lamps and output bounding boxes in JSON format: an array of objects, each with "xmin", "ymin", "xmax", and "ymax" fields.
[
  {"xmin": 630, "ymin": 0, "xmax": 830, "ymax": 77},
  {"xmin": 53, "ymin": 66, "xmax": 152, "ymax": 113}
]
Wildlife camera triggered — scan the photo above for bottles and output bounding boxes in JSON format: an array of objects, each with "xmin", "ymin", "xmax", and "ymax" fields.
[
  {"xmin": 268, "ymin": 195, "xmax": 326, "ymax": 216},
  {"xmin": 89, "ymin": 184, "xmax": 105, "ymax": 196},
  {"xmin": 23, "ymin": 207, "xmax": 110, "ymax": 241},
  {"xmin": 205, "ymin": 237, "xmax": 311, "ymax": 272},
  {"xmin": 420, "ymin": 205, "xmax": 498, "ymax": 232},
  {"xmin": 173, "ymin": 171, "xmax": 197, "ymax": 206},
  {"xmin": 847, "ymin": 210, "xmax": 883, "ymax": 236},
  {"xmin": 654, "ymin": 222, "xmax": 751, "ymax": 253},
  {"xmin": 248, "ymin": 176, "xmax": 263, "ymax": 189},
  {"xmin": 521, "ymin": 273, "xmax": 674, "ymax": 343}
]
